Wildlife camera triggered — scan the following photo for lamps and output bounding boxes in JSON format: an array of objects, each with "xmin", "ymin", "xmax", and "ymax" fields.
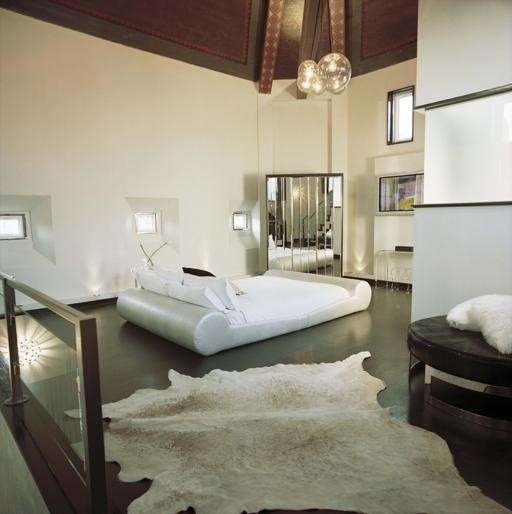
[{"xmin": 297, "ymin": 52, "xmax": 352, "ymax": 95}]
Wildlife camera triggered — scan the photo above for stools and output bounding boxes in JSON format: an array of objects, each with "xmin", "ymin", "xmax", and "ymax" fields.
[{"xmin": 391, "ymin": 267, "xmax": 413, "ymax": 294}]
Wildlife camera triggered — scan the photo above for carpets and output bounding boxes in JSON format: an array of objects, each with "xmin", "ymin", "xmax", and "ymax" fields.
[{"xmin": 64, "ymin": 350, "xmax": 511, "ymax": 514}]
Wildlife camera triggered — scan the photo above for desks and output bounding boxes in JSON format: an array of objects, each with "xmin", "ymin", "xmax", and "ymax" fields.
[
  {"xmin": 408, "ymin": 315, "xmax": 512, "ymax": 432},
  {"xmin": 375, "ymin": 249, "xmax": 414, "ymax": 290}
]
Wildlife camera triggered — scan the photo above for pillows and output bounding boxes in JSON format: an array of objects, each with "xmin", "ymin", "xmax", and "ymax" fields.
[
  {"xmin": 269, "ymin": 234, "xmax": 275, "ymax": 249},
  {"xmin": 445, "ymin": 294, "xmax": 512, "ymax": 354},
  {"xmin": 131, "ymin": 265, "xmax": 243, "ymax": 313}
]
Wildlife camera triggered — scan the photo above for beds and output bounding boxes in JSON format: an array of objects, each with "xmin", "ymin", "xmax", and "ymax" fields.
[
  {"xmin": 117, "ymin": 268, "xmax": 372, "ymax": 357},
  {"xmin": 268, "ymin": 246, "xmax": 333, "ymax": 272}
]
